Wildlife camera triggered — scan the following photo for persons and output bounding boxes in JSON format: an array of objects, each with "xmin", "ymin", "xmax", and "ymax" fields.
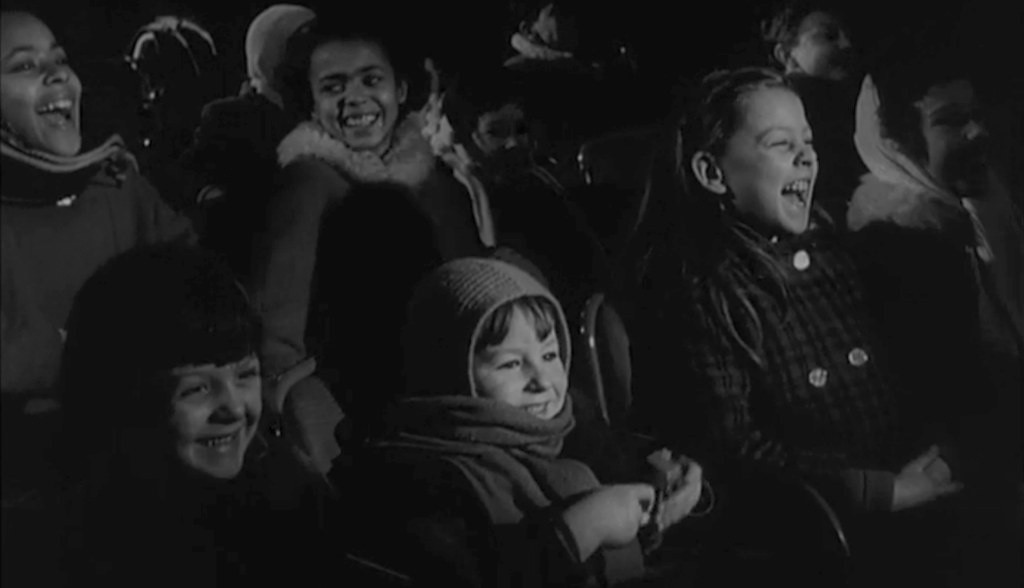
[
  {"xmin": 254, "ymin": 14, "xmax": 497, "ymax": 478},
  {"xmin": 674, "ymin": 0, "xmax": 1024, "ymax": 588},
  {"xmin": 1, "ymin": 3, "xmax": 317, "ymax": 420},
  {"xmin": 464, "ymin": 1, "xmax": 585, "ymax": 193},
  {"xmin": 1, "ymin": 242, "xmax": 705, "ymax": 588}
]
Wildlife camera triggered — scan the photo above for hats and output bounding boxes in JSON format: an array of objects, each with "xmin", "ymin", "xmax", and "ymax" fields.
[
  {"xmin": 245, "ymin": 5, "xmax": 317, "ymax": 103},
  {"xmin": 405, "ymin": 252, "xmax": 571, "ymax": 404}
]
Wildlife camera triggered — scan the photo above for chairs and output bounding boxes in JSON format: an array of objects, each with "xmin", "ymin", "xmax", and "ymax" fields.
[{"xmin": 197, "ymin": 123, "xmax": 858, "ymax": 559}]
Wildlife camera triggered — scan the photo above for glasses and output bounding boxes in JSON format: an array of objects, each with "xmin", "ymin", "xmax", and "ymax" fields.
[{"xmin": 699, "ymin": 65, "xmax": 785, "ymax": 110}]
